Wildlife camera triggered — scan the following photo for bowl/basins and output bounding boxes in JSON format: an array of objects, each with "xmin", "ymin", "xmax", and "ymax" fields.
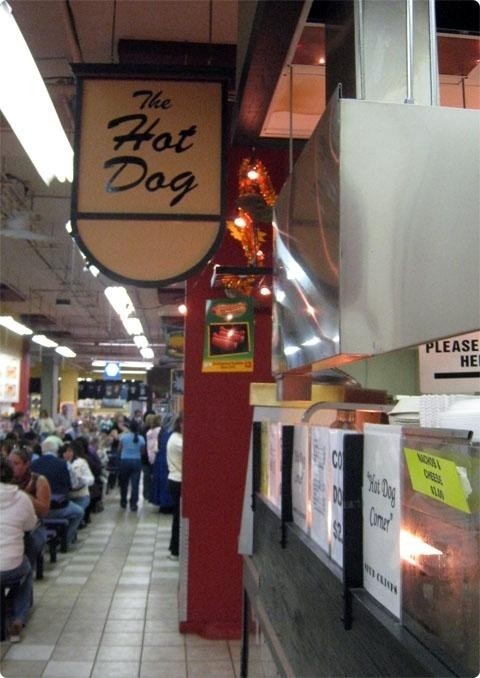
[{"xmin": 354, "ymin": 410, "xmax": 382, "ymax": 434}]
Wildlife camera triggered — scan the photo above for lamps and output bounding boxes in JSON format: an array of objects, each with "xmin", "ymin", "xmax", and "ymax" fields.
[
  {"xmin": 227, "ymin": 209, "xmax": 270, "ymax": 266},
  {"xmin": 219, "ymin": 276, "xmax": 255, "ymax": 296},
  {"xmin": 238, "ymin": 141, "xmax": 278, "ymax": 205}
]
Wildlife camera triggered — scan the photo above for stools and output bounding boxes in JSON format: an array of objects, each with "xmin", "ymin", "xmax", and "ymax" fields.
[
  {"xmin": 36, "ymin": 537, "xmax": 42, "ymax": 579},
  {"xmin": 0, "ymin": 579, "xmax": 18, "ymax": 644},
  {"xmin": 44, "ymin": 517, "xmax": 69, "ymax": 552},
  {"xmin": 45, "ymin": 529, "xmax": 58, "ymax": 563}
]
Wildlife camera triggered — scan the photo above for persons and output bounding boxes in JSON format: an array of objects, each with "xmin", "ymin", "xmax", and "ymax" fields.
[
  {"xmin": 108, "ymin": 413, "xmax": 130, "ymax": 489},
  {"xmin": 145, "ymin": 414, "xmax": 163, "ymax": 504},
  {"xmin": 140, "ymin": 411, "xmax": 156, "ymax": 499},
  {"xmin": 133, "ymin": 409, "xmax": 142, "ymax": 423},
  {"xmin": 31, "ymin": 437, "xmax": 84, "ymax": 546},
  {"xmin": 10, "ymin": 410, "xmax": 26, "ymax": 437},
  {"xmin": 75, "ymin": 436, "xmax": 104, "ymax": 513},
  {"xmin": 62, "ymin": 434, "xmax": 73, "ymax": 444},
  {"xmin": 166, "ymin": 414, "xmax": 182, "ymax": 561},
  {"xmin": 36, "ymin": 410, "xmax": 54, "ymax": 434},
  {"xmin": 41, "ymin": 426, "xmax": 65, "ymax": 456},
  {"xmin": 0, "ymin": 455, "xmax": 39, "ymax": 636},
  {"xmin": 23, "ymin": 431, "xmax": 39, "ymax": 449},
  {"xmin": 74, "ymin": 412, "xmax": 111, "ymax": 435},
  {"xmin": 55, "ymin": 405, "xmax": 73, "ymax": 433},
  {"xmin": 59, "ymin": 444, "xmax": 95, "ymax": 529},
  {"xmin": 153, "ymin": 415, "xmax": 172, "ymax": 515},
  {"xmin": 117, "ymin": 419, "xmax": 146, "ymax": 511},
  {"xmin": 7, "ymin": 446, "xmax": 51, "ymax": 570},
  {"xmin": 0, "ymin": 433, "xmax": 16, "ymax": 459}
]
